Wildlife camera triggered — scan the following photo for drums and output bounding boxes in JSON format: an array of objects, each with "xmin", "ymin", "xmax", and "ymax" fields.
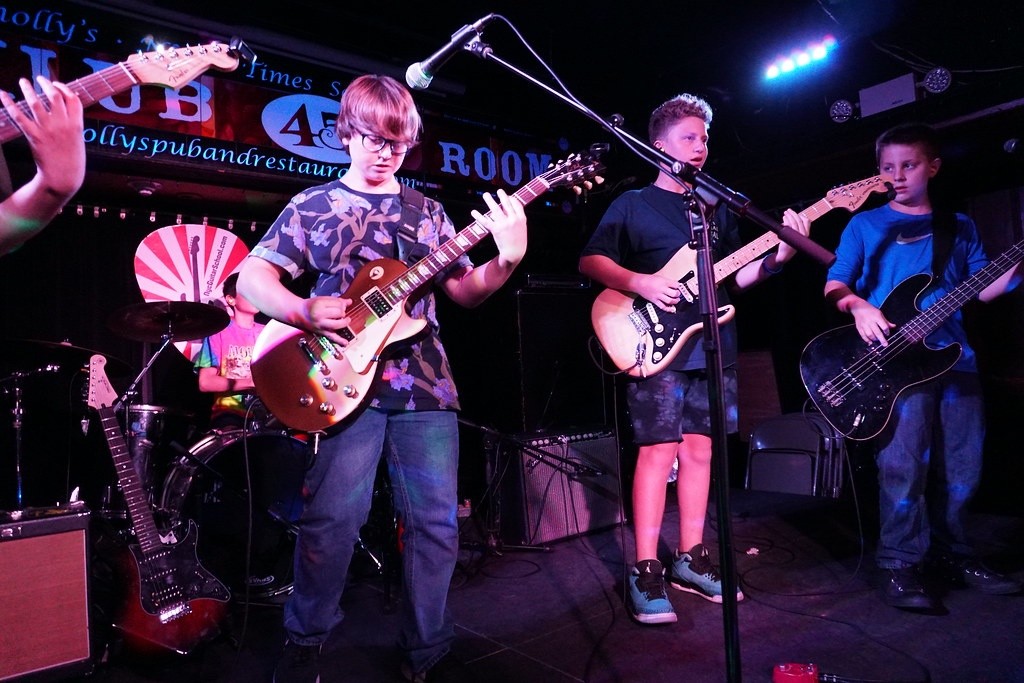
[
  {"xmin": 147, "ymin": 426, "xmax": 329, "ymax": 609},
  {"xmin": 92, "ymin": 401, "xmax": 170, "ymax": 526}
]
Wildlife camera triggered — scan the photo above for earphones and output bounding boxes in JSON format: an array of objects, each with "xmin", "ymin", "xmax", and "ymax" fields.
[
  {"xmin": 661, "ymin": 148, "xmax": 665, "ymax": 152},
  {"xmin": 932, "ymin": 168, "xmax": 936, "ymax": 172}
]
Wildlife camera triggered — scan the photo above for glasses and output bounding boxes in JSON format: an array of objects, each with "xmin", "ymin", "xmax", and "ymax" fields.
[{"xmin": 347, "ymin": 121, "xmax": 409, "ymax": 154}]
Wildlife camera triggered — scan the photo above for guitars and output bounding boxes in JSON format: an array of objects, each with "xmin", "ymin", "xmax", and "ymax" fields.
[
  {"xmin": 78, "ymin": 352, "xmax": 233, "ymax": 666},
  {"xmin": 250, "ymin": 140, "xmax": 614, "ymax": 440},
  {"xmin": 589, "ymin": 175, "xmax": 890, "ymax": 382},
  {"xmin": 0, "ymin": 39, "xmax": 237, "ymax": 146},
  {"xmin": 798, "ymin": 241, "xmax": 1024, "ymax": 443}
]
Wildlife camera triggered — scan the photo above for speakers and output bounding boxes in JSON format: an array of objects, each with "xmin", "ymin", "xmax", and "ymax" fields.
[
  {"xmin": 0, "ymin": 509, "xmax": 95, "ymax": 683},
  {"xmin": 508, "ymin": 287, "xmax": 607, "ymax": 433},
  {"xmin": 490, "ymin": 429, "xmax": 632, "ymax": 544}
]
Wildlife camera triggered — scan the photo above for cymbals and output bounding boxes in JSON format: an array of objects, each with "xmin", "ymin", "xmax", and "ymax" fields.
[
  {"xmin": 111, "ymin": 301, "xmax": 231, "ymax": 342},
  {"xmin": 22, "ymin": 338, "xmax": 132, "ymax": 375}
]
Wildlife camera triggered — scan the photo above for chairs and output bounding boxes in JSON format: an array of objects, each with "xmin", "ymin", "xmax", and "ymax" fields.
[{"xmin": 744, "ymin": 413, "xmax": 847, "ymax": 499}]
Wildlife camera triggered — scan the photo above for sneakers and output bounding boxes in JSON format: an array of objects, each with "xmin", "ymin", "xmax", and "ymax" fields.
[
  {"xmin": 875, "ymin": 567, "xmax": 934, "ymax": 607},
  {"xmin": 670, "ymin": 542, "xmax": 744, "ymax": 603},
  {"xmin": 401, "ymin": 649, "xmax": 479, "ymax": 683},
  {"xmin": 627, "ymin": 559, "xmax": 678, "ymax": 624},
  {"xmin": 934, "ymin": 551, "xmax": 1021, "ymax": 594},
  {"xmin": 273, "ymin": 639, "xmax": 322, "ymax": 683}
]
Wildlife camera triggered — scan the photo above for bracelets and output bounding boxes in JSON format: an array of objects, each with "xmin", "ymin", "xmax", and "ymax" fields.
[{"xmin": 762, "ymin": 253, "xmax": 785, "ymax": 275}]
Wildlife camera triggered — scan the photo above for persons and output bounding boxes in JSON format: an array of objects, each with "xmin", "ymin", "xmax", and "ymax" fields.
[
  {"xmin": 0, "ymin": 74, "xmax": 88, "ymax": 253},
  {"xmin": 235, "ymin": 73, "xmax": 529, "ymax": 683},
  {"xmin": 823, "ymin": 121, "xmax": 1024, "ymax": 611},
  {"xmin": 196, "ymin": 275, "xmax": 269, "ymax": 597},
  {"xmin": 578, "ymin": 92, "xmax": 811, "ymax": 624}
]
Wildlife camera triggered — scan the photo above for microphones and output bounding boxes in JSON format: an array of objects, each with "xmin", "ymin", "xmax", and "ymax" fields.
[
  {"xmin": 1004, "ymin": 139, "xmax": 1024, "ymax": 154},
  {"xmin": 576, "ymin": 469, "xmax": 606, "ymax": 478},
  {"xmin": 405, "ymin": 13, "xmax": 495, "ymax": 90}
]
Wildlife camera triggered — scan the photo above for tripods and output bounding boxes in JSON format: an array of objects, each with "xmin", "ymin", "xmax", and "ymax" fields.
[{"xmin": 457, "ymin": 419, "xmax": 590, "ymax": 580}]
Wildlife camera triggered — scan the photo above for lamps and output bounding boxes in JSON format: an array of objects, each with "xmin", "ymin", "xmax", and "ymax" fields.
[{"xmin": 829, "ymin": 67, "xmax": 952, "ymax": 123}]
